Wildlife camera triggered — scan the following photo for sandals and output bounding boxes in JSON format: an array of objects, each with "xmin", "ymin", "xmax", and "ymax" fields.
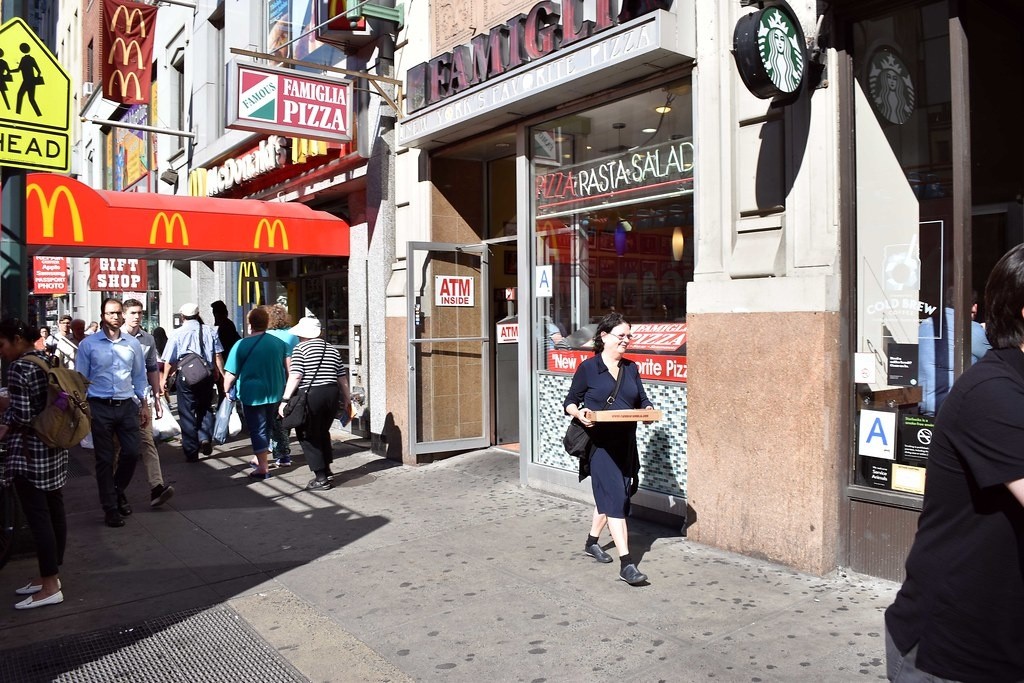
[{"xmin": 305, "ymin": 475, "xmax": 333, "ymax": 491}]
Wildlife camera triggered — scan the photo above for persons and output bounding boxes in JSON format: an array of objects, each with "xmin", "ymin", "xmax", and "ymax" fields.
[
  {"xmin": 535, "ymin": 314, "xmax": 566, "ymax": 371},
  {"xmin": 917, "ymin": 280, "xmax": 993, "ymax": 417},
  {"xmin": 561, "ymin": 311, "xmax": 653, "ymax": 583},
  {"xmin": 0, "ymin": 311, "xmax": 79, "ymax": 610},
  {"xmin": 112, "ymin": 298, "xmax": 176, "ymax": 514},
  {"xmin": 277, "ymin": 316, "xmax": 353, "ymax": 490},
  {"xmin": 74, "ymin": 299, "xmax": 152, "ymax": 528},
  {"xmin": 33, "ymin": 299, "xmax": 304, "ymax": 481},
  {"xmin": 882, "ymin": 242, "xmax": 1024, "ymax": 683}
]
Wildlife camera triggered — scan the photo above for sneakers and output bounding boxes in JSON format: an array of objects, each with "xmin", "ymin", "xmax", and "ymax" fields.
[
  {"xmin": 151, "ymin": 483, "xmax": 174, "ymax": 507},
  {"xmin": 275, "ymin": 457, "xmax": 293, "ymax": 466}
]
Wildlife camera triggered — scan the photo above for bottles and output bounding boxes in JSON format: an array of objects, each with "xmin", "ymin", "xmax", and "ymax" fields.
[{"xmin": 52, "ymin": 392, "xmax": 70, "ymax": 412}]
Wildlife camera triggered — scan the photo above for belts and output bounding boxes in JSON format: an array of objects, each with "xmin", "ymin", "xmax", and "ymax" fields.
[{"xmin": 89, "ymin": 397, "xmax": 122, "ymax": 407}]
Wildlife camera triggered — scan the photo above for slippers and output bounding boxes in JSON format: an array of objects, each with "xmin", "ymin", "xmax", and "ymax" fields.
[
  {"xmin": 250, "ymin": 470, "xmax": 271, "ymax": 479},
  {"xmin": 249, "ymin": 461, "xmax": 259, "ymax": 468}
]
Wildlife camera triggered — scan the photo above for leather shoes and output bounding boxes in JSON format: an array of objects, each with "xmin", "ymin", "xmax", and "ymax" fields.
[
  {"xmin": 620, "ymin": 564, "xmax": 648, "ymax": 583},
  {"xmin": 585, "ymin": 543, "xmax": 612, "ymax": 562},
  {"xmin": 106, "ymin": 513, "xmax": 125, "ymax": 527},
  {"xmin": 119, "ymin": 500, "xmax": 132, "ymax": 517}
]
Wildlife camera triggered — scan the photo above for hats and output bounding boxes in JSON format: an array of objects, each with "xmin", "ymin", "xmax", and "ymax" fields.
[
  {"xmin": 179, "ymin": 304, "xmax": 199, "ymax": 316},
  {"xmin": 288, "ymin": 318, "xmax": 321, "ymax": 338}
]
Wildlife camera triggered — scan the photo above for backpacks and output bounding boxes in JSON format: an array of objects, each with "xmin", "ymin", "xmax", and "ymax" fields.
[
  {"xmin": 177, "ymin": 323, "xmax": 212, "ymax": 385},
  {"xmin": 20, "ymin": 355, "xmax": 93, "ymax": 449}
]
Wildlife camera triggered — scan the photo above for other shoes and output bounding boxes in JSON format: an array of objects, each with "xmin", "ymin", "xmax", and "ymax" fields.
[
  {"xmin": 15, "ymin": 591, "xmax": 63, "ymax": 609},
  {"xmin": 201, "ymin": 440, "xmax": 213, "ymax": 456},
  {"xmin": 168, "ymin": 405, "xmax": 174, "ymax": 409},
  {"xmin": 185, "ymin": 457, "xmax": 198, "ymax": 464},
  {"xmin": 16, "ymin": 578, "xmax": 62, "ymax": 594}
]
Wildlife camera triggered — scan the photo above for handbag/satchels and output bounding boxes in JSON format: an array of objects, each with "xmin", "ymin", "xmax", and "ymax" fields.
[
  {"xmin": 149, "ymin": 397, "xmax": 182, "ymax": 443},
  {"xmin": 212, "ymin": 385, "xmax": 242, "ymax": 445},
  {"xmin": 282, "ymin": 394, "xmax": 311, "ymax": 428},
  {"xmin": 563, "ymin": 417, "xmax": 593, "ymax": 459},
  {"xmin": 167, "ymin": 365, "xmax": 178, "ymax": 392}
]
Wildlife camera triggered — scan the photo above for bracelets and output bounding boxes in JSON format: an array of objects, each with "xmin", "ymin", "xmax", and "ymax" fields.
[
  {"xmin": 281, "ymin": 398, "xmax": 290, "ymax": 403},
  {"xmin": 224, "ymin": 391, "xmax": 229, "ymax": 394}
]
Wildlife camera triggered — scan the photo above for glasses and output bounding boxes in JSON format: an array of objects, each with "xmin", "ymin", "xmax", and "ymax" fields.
[
  {"xmin": 607, "ymin": 332, "xmax": 633, "ymax": 340},
  {"xmin": 104, "ymin": 311, "xmax": 123, "ymax": 317}
]
